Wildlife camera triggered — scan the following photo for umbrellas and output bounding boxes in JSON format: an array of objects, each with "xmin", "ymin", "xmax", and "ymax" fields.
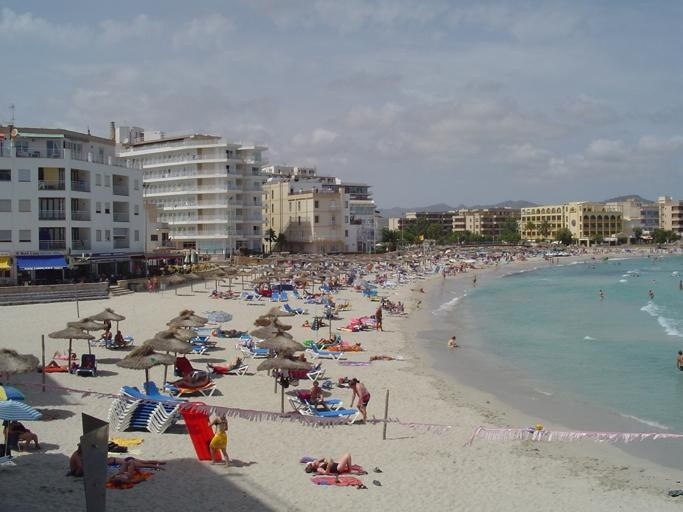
[
  {"xmin": 0, "ymin": 398, "xmax": 44, "ymax": 456},
  {"xmin": 251, "ymin": 303, "xmax": 312, "ymax": 413},
  {"xmin": 115, "ymin": 343, "xmax": 176, "ymax": 385},
  {"xmin": 206, "ymin": 309, "xmax": 233, "ymax": 323},
  {"xmin": 82, "ymin": 307, "xmax": 125, "ymax": 334},
  {"xmin": 0, "ymin": 382, "xmax": 27, "ymax": 401},
  {"xmin": 142, "ymin": 308, "xmax": 208, "ymax": 392},
  {"xmin": 66, "ymin": 317, "xmax": 107, "ymax": 354},
  {"xmin": 163, "ymin": 252, "xmax": 351, "ymax": 298},
  {"xmin": 47, "ymin": 326, "xmax": 94, "ymax": 367}
]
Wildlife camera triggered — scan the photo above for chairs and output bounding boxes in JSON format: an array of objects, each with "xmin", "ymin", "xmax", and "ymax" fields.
[
  {"xmin": 285, "ymin": 396, "xmax": 342, "ymax": 417},
  {"xmin": 107, "ymin": 381, "xmax": 185, "ymax": 434},
  {"xmin": 179, "ymin": 401, "xmax": 222, "ymax": 460},
  {"xmin": 77, "ymin": 354, "xmax": 97, "ymax": 377},
  {"xmin": 298, "ymin": 399, "xmax": 360, "ymax": 426},
  {"xmin": 165, "ymin": 379, "xmax": 219, "ymax": 397},
  {"xmin": 159, "ymin": 261, "xmax": 398, "ymax": 375},
  {"xmin": 92, "ymin": 336, "xmax": 134, "ymax": 348}
]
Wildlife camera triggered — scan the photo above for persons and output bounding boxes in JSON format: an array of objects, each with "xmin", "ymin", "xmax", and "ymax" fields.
[
  {"xmin": 675, "ymin": 349, "xmax": 683, "ymax": 371},
  {"xmin": 94, "ymin": 329, "xmax": 112, "ymax": 343},
  {"xmin": 304, "ymin": 454, "xmax": 365, "ymax": 476},
  {"xmin": 108, "ymin": 459, "xmax": 153, "ymax": 487},
  {"xmin": 374, "ymin": 305, "xmax": 384, "ymax": 332},
  {"xmin": 446, "ymin": 335, "xmax": 457, "ymax": 349},
  {"xmin": 348, "ymin": 376, "xmax": 372, "ymax": 423},
  {"xmin": 50, "ymin": 350, "xmax": 77, "ymax": 360},
  {"xmin": 145, "ymin": 272, "xmax": 161, "ymax": 294},
  {"xmin": 8, "ymin": 420, "xmax": 41, "ymax": 450},
  {"xmin": 379, "ymin": 296, "xmax": 406, "ymax": 314},
  {"xmin": 206, "ymin": 411, "xmax": 232, "ymax": 469},
  {"xmin": 81, "ymin": 356, "xmax": 92, "ymax": 368},
  {"xmin": 68, "ymin": 441, "xmax": 83, "ymax": 477},
  {"xmin": 114, "ymin": 331, "xmax": 126, "ymax": 349},
  {"xmin": 308, "ymin": 380, "xmax": 330, "ymax": 411},
  {"xmin": 205, "ymin": 357, "xmax": 242, "ymax": 373},
  {"xmin": 106, "ymin": 455, "xmax": 167, "ymax": 470},
  {"xmin": 210, "ymin": 326, "xmax": 254, "ymax": 349},
  {"xmin": 43, "ymin": 360, "xmax": 75, "ymax": 370},
  {"xmin": 0, "ymin": 418, "xmax": 12, "ymax": 458},
  {"xmin": 316, "ymin": 332, "xmax": 363, "ymax": 352}
]
